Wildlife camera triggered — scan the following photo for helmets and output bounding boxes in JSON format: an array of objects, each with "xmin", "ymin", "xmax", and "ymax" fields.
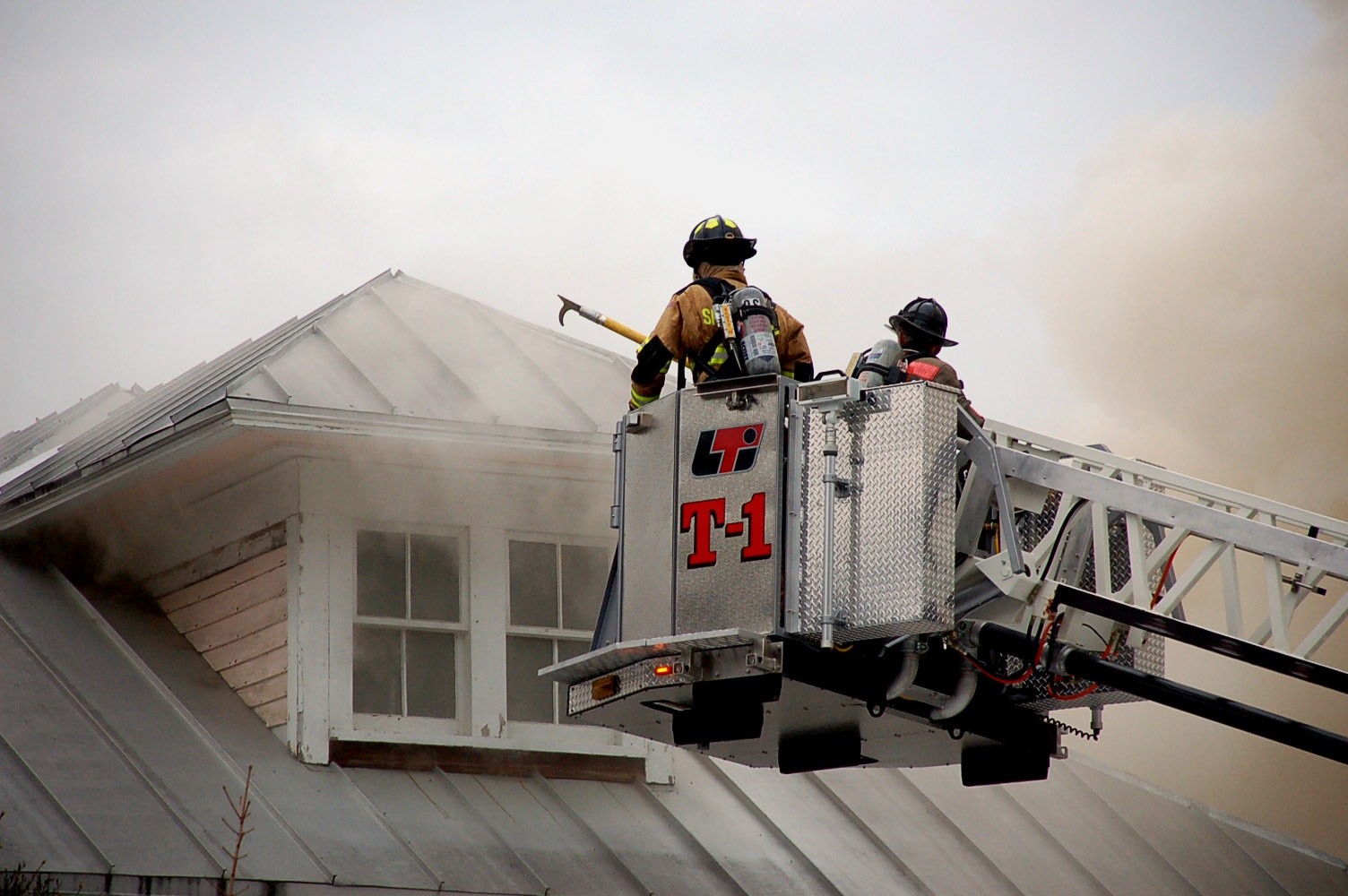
[
  {"xmin": 683, "ymin": 215, "xmax": 757, "ymax": 268},
  {"xmin": 889, "ymin": 299, "xmax": 958, "ymax": 347}
]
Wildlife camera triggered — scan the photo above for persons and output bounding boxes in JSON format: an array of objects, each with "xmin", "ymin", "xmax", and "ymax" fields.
[
  {"xmin": 628, "ymin": 214, "xmax": 814, "ymax": 412},
  {"xmin": 847, "ymin": 297, "xmax": 985, "ymax": 448}
]
